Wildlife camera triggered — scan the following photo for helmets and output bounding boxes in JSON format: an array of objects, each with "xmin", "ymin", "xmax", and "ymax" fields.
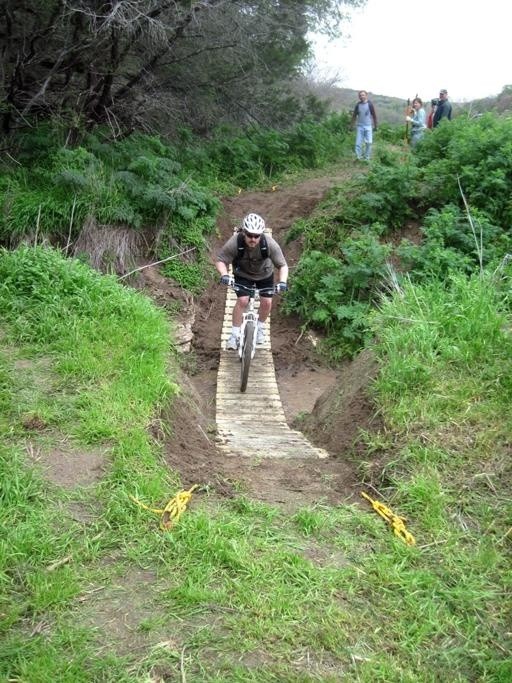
[{"xmin": 241, "ymin": 213, "xmax": 265, "ymax": 234}]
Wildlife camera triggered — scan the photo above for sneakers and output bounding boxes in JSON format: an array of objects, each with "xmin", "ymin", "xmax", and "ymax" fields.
[
  {"xmin": 226, "ymin": 335, "xmax": 240, "ymax": 350},
  {"xmin": 256, "ymin": 327, "xmax": 264, "ymax": 344}
]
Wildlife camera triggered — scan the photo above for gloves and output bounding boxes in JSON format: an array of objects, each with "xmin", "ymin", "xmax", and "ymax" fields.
[
  {"xmin": 220, "ymin": 274, "xmax": 235, "ymax": 288},
  {"xmin": 273, "ymin": 281, "xmax": 287, "ymax": 295}
]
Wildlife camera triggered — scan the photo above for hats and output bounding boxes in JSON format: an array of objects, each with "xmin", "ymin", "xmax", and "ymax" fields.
[{"xmin": 439, "ymin": 89, "xmax": 447, "ymax": 94}]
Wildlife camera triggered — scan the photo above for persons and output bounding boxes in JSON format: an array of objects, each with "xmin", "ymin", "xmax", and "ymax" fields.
[
  {"xmin": 351, "ymin": 89, "xmax": 378, "ymax": 162},
  {"xmin": 405, "ymin": 97, "xmax": 426, "ymax": 135},
  {"xmin": 214, "ymin": 211, "xmax": 290, "ymax": 351},
  {"xmin": 432, "ymin": 88, "xmax": 452, "ymax": 126},
  {"xmin": 428, "ymin": 97, "xmax": 439, "ymax": 126}
]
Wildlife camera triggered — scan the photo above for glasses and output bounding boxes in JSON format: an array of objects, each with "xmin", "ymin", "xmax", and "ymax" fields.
[{"xmin": 244, "ymin": 230, "xmax": 261, "ymax": 238}]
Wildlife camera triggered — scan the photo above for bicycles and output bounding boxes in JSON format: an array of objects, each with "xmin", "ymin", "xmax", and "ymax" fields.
[{"xmin": 230, "ymin": 281, "xmax": 273, "ymax": 391}]
